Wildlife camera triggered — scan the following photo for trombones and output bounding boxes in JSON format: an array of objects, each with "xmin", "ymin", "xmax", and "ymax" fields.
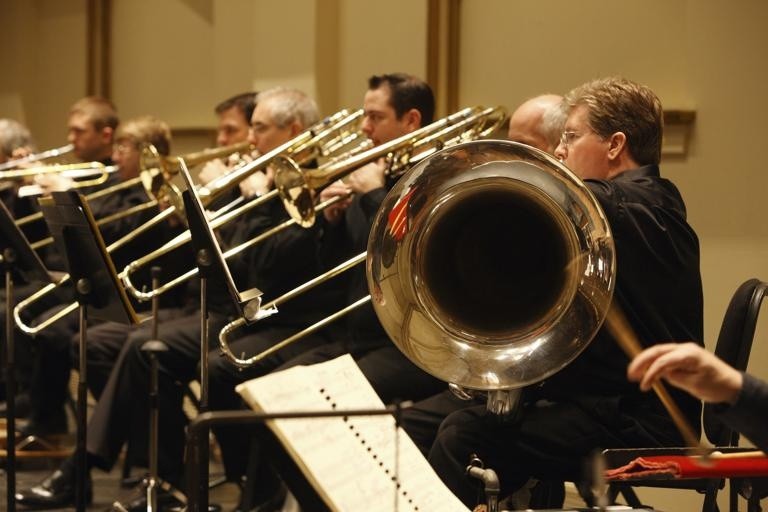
[
  {"xmin": 122, "ymin": 111, "xmax": 359, "ymax": 303},
  {"xmin": 0, "ymin": 143, "xmax": 122, "ymax": 199},
  {"xmin": 217, "ymin": 106, "xmax": 513, "ymax": 364},
  {"xmin": 10, "ymin": 139, "xmax": 267, "ymax": 335}
]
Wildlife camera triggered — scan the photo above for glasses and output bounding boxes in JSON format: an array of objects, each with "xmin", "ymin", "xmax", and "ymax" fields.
[{"xmin": 559, "ymin": 130, "xmax": 614, "ymax": 144}]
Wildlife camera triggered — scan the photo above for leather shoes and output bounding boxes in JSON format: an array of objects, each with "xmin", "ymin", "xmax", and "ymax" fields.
[
  {"xmin": 16, "ymin": 469, "xmax": 92, "ymax": 508},
  {"xmin": 0, "ymin": 395, "xmax": 30, "ymax": 419},
  {"xmin": 18, "ymin": 421, "xmax": 71, "ymax": 436}
]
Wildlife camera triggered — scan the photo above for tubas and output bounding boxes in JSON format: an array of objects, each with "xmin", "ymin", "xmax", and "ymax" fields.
[{"xmin": 366, "ymin": 136, "xmax": 616, "ymax": 409}]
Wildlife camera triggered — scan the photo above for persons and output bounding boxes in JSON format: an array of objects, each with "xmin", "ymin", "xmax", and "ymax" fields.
[
  {"xmin": 3, "ymin": 71, "xmax": 707, "ymax": 511},
  {"xmin": 622, "ymin": 338, "xmax": 768, "ymax": 456}
]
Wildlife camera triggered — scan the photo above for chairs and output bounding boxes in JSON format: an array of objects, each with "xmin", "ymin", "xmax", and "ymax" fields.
[{"xmin": 604, "ymin": 277, "xmax": 768, "ymax": 510}]
